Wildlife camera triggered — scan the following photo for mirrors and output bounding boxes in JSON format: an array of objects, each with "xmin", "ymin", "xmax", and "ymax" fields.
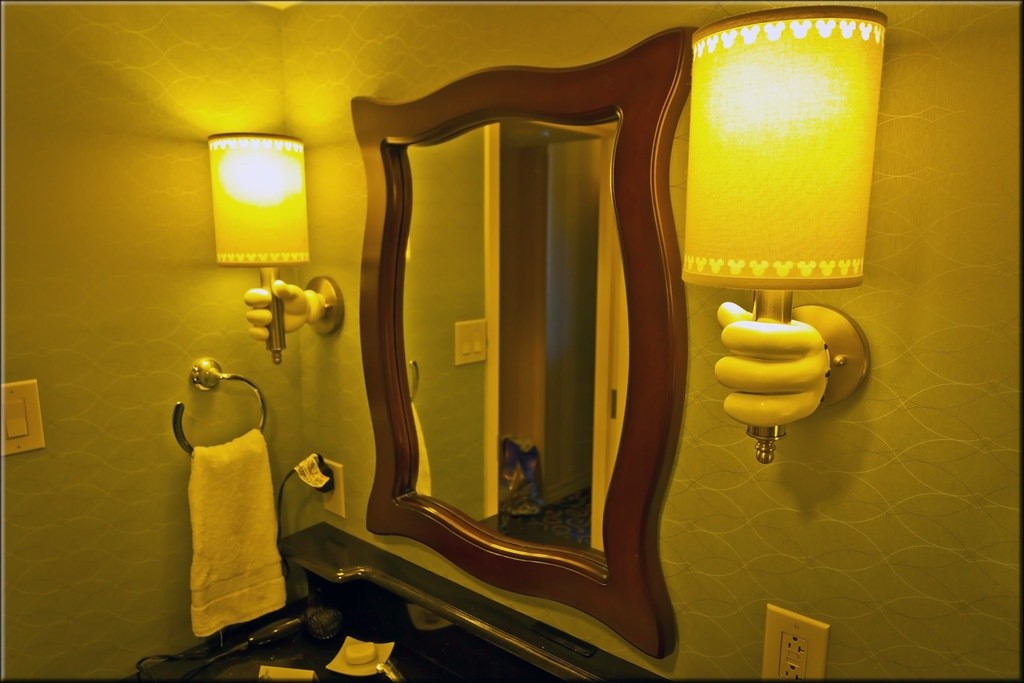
[{"xmin": 345, "ymin": 24, "xmax": 698, "ymax": 660}]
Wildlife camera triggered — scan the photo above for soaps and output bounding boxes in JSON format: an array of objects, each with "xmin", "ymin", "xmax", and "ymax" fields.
[{"xmin": 346, "ymin": 643, "xmax": 376, "ymax": 665}]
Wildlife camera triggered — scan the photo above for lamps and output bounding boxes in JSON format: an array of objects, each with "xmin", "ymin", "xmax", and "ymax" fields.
[
  {"xmin": 678, "ymin": 5, "xmax": 890, "ymax": 464},
  {"xmin": 206, "ymin": 132, "xmax": 346, "ymax": 366}
]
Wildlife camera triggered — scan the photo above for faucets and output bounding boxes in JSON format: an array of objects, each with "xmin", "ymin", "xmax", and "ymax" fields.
[{"xmin": 376, "ymin": 662, "xmax": 408, "ymax": 683}]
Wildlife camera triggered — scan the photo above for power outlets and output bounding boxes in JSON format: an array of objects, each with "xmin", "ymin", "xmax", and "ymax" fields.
[
  {"xmin": 761, "ymin": 602, "xmax": 832, "ymax": 680},
  {"xmin": 322, "ymin": 458, "xmax": 345, "ymax": 518}
]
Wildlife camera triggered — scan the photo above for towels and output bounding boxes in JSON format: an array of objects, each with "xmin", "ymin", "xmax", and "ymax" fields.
[
  {"xmin": 187, "ymin": 429, "xmax": 287, "ymax": 636},
  {"xmin": 409, "ymin": 401, "xmax": 431, "ymax": 497}
]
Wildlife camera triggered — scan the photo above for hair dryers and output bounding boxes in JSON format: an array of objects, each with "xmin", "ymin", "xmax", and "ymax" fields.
[{"xmin": 248, "ymin": 608, "xmax": 343, "ymax": 648}]
